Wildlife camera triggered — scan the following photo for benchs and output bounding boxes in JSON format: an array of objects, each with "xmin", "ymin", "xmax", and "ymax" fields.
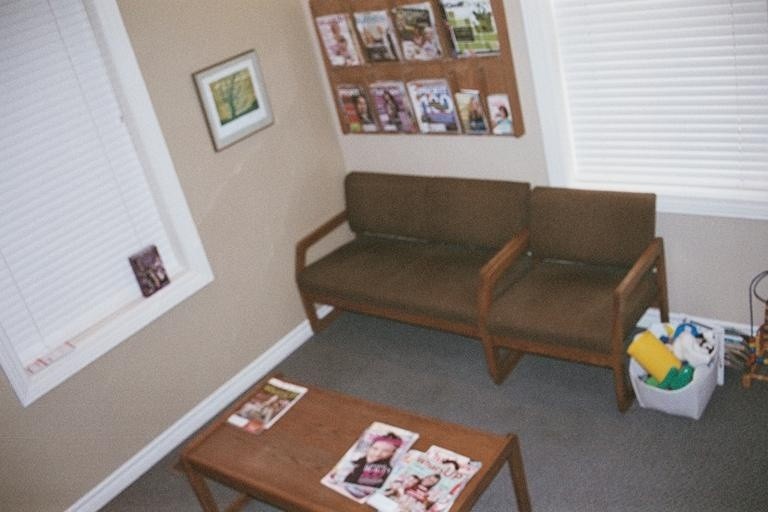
[{"xmin": 293, "ymin": 170, "xmax": 531, "ymax": 389}]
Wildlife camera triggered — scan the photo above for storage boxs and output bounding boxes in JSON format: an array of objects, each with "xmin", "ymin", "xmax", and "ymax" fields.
[{"xmin": 628, "ymin": 320, "xmax": 726, "ymax": 421}]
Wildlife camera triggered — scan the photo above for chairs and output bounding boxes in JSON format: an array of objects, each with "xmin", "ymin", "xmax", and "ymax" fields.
[{"xmin": 485, "ymin": 183, "xmax": 671, "ymax": 413}]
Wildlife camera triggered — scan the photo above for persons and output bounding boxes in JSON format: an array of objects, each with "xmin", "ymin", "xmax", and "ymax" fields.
[{"xmin": 239, "ymin": 395, "xmax": 279, "ymax": 426}]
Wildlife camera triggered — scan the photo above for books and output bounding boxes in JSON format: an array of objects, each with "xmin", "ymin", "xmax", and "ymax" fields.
[
  {"xmin": 225, "ymin": 375, "xmax": 308, "ymax": 435},
  {"xmin": 129, "ymin": 244, "xmax": 170, "ymax": 297},
  {"xmin": 315, "ymin": 0, "xmax": 515, "ymax": 135},
  {"xmin": 320, "ymin": 420, "xmax": 482, "ymax": 512}
]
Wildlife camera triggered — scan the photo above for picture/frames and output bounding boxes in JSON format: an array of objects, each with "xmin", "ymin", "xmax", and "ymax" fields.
[{"xmin": 190, "ymin": 47, "xmax": 276, "ymax": 155}]
